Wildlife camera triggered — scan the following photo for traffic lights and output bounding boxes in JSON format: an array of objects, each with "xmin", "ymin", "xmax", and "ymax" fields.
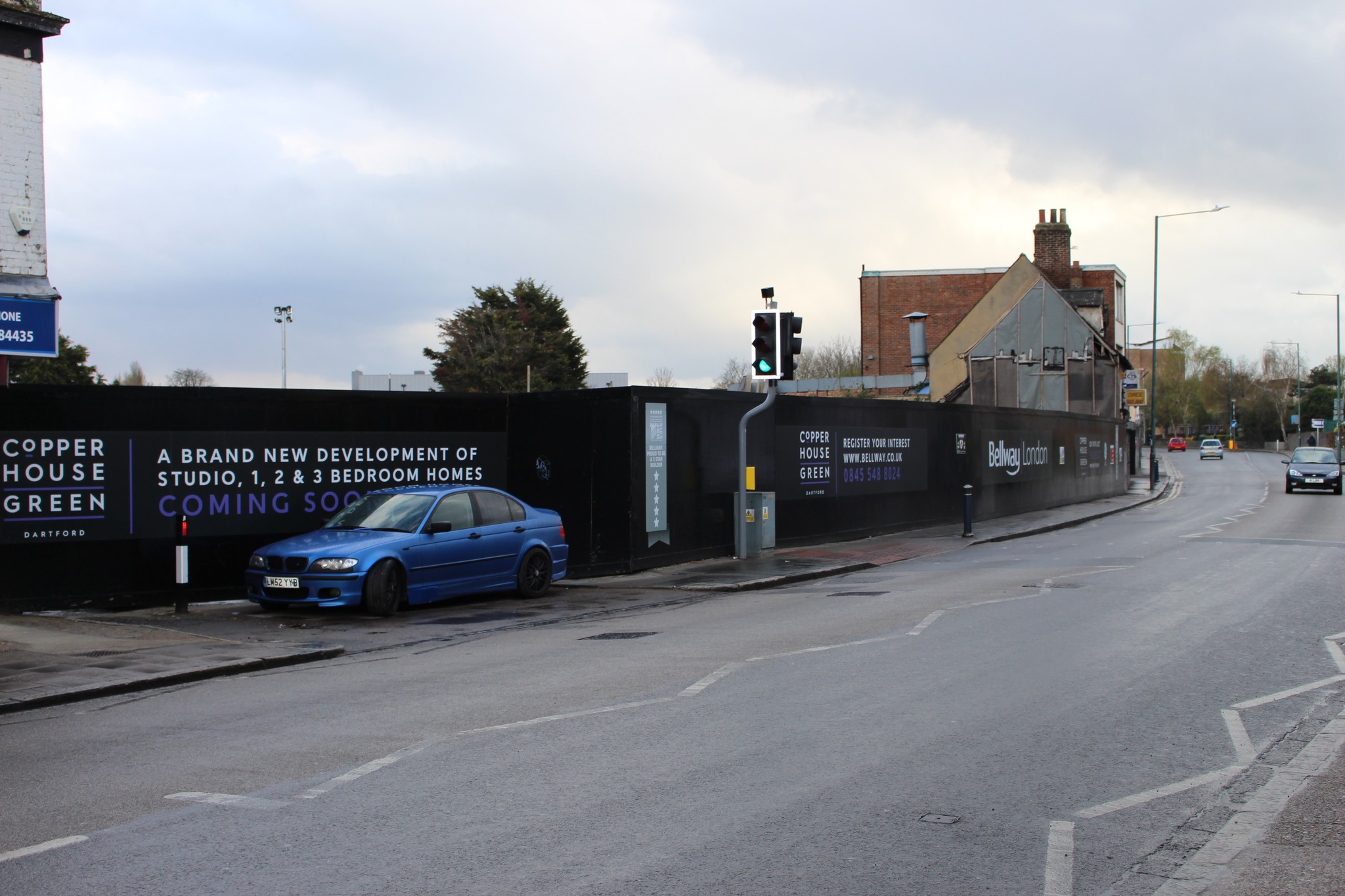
[
  {"xmin": 782, "ymin": 312, "xmax": 802, "ymax": 380},
  {"xmin": 752, "ymin": 312, "xmax": 779, "ymax": 380}
]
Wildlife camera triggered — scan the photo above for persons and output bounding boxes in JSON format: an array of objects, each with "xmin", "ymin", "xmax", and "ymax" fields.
[
  {"xmin": 1307, "ymin": 435, "xmax": 1316, "ymax": 446},
  {"xmin": 1213, "ymin": 434, "xmax": 1217, "ymax": 438}
]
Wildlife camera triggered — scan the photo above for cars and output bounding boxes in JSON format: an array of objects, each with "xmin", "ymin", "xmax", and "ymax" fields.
[
  {"xmin": 1281, "ymin": 447, "xmax": 1345, "ymax": 496},
  {"xmin": 247, "ymin": 484, "xmax": 570, "ymax": 617},
  {"xmin": 1200, "ymin": 439, "xmax": 1225, "ymax": 460},
  {"xmin": 1166, "ymin": 437, "xmax": 1186, "ymax": 452}
]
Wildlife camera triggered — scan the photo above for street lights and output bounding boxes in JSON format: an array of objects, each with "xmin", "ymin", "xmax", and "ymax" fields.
[
  {"xmin": 1291, "ymin": 291, "xmax": 1342, "ymax": 477},
  {"xmin": 1127, "ymin": 322, "xmax": 1164, "ymax": 360},
  {"xmin": 1266, "ymin": 341, "xmax": 1301, "ymax": 448},
  {"xmin": 275, "ymin": 306, "xmax": 292, "ymax": 389},
  {"xmin": 1150, "ymin": 205, "xmax": 1232, "ymax": 492},
  {"xmin": 1207, "ymin": 357, "xmax": 1232, "ymax": 440}
]
